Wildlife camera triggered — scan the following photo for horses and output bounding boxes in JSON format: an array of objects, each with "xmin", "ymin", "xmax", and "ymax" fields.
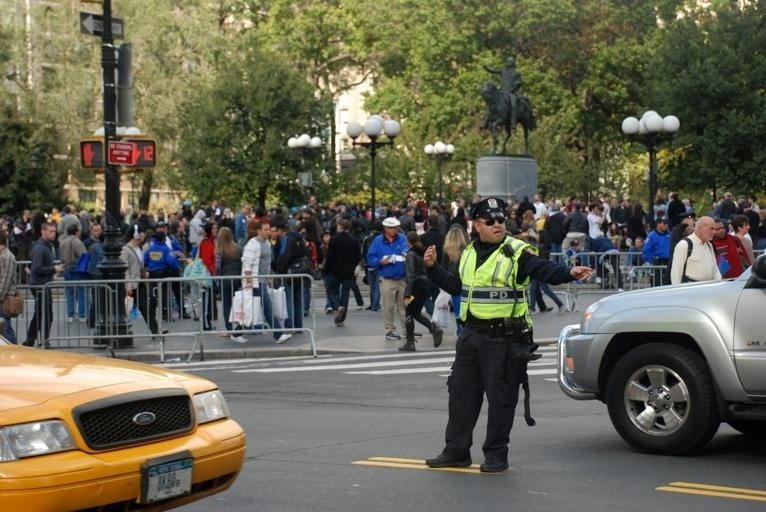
[{"xmin": 479, "ymin": 82, "xmax": 539, "ymax": 157}]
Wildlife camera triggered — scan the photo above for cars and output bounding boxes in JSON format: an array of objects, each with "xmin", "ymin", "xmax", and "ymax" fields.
[{"xmin": 0, "ymin": 332, "xmax": 246, "ymax": 512}]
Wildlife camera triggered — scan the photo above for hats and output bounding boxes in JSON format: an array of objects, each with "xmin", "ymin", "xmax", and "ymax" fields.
[
  {"xmin": 571, "ymin": 240, "xmax": 580, "ymax": 245},
  {"xmin": 380, "ymin": 217, "xmax": 402, "ymax": 228},
  {"xmin": 468, "ymin": 196, "xmax": 507, "ymax": 220},
  {"xmin": 655, "ymin": 215, "xmax": 671, "ymax": 222}
]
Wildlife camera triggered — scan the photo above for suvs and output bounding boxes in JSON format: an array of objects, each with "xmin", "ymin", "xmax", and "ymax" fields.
[{"xmin": 556, "ymin": 245, "xmax": 766, "ymax": 452}]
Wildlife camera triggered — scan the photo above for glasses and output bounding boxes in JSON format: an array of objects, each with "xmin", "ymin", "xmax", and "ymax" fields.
[{"xmin": 475, "ymin": 218, "xmax": 506, "ymax": 227}]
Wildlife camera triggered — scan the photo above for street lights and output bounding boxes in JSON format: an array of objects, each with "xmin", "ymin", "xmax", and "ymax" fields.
[
  {"xmin": 346, "ymin": 113, "xmax": 402, "ymax": 226},
  {"xmin": 283, "ymin": 134, "xmax": 325, "ymax": 214},
  {"xmin": 621, "ymin": 110, "xmax": 684, "ymax": 232},
  {"xmin": 420, "ymin": 141, "xmax": 458, "ymax": 215}
]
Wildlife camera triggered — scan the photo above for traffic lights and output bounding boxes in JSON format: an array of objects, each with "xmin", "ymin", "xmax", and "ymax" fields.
[
  {"xmin": 125, "ymin": 137, "xmax": 157, "ymax": 170},
  {"xmin": 76, "ymin": 137, "xmax": 107, "ymax": 168}
]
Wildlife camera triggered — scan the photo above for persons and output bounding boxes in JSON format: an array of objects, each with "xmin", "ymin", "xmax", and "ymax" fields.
[{"xmin": 423, "ymin": 195, "xmax": 593, "ymax": 471}]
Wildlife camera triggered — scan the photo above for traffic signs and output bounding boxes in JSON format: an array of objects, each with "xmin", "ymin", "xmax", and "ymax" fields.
[
  {"xmin": 73, "ymin": 11, "xmax": 130, "ymax": 42},
  {"xmin": 107, "ymin": 140, "xmax": 138, "ymax": 168}
]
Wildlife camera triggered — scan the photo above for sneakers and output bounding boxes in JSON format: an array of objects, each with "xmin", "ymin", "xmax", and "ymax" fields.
[
  {"xmin": 402, "ymin": 332, "xmax": 423, "ymax": 340},
  {"xmin": 423, "ymin": 443, "xmax": 473, "ymax": 469},
  {"xmin": 385, "ymin": 330, "xmax": 401, "ymax": 341},
  {"xmin": 276, "ymin": 333, "xmax": 294, "ymax": 345},
  {"xmin": 397, "ymin": 343, "xmax": 417, "ymax": 353},
  {"xmin": 152, "ymin": 309, "xmax": 226, "ymax": 342},
  {"xmin": 432, "ymin": 327, "xmax": 443, "ymax": 347},
  {"xmin": 324, "ymin": 301, "xmax": 383, "ymax": 328},
  {"xmin": 66, "ymin": 314, "xmax": 88, "ymax": 325},
  {"xmin": 229, "ymin": 334, "xmax": 249, "ymax": 344},
  {"xmin": 479, "ymin": 445, "xmax": 511, "ymax": 473},
  {"xmin": 526, "ymin": 273, "xmax": 626, "ymax": 316}
]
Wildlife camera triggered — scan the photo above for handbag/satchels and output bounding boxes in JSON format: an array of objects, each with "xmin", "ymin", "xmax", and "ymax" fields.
[
  {"xmin": 266, "ymin": 285, "xmax": 290, "ymax": 322},
  {"xmin": 2, "ymin": 290, "xmax": 27, "ymax": 319},
  {"xmin": 228, "ymin": 288, "xmax": 265, "ymax": 328},
  {"xmin": 286, "ymin": 254, "xmax": 312, "ymax": 277}
]
